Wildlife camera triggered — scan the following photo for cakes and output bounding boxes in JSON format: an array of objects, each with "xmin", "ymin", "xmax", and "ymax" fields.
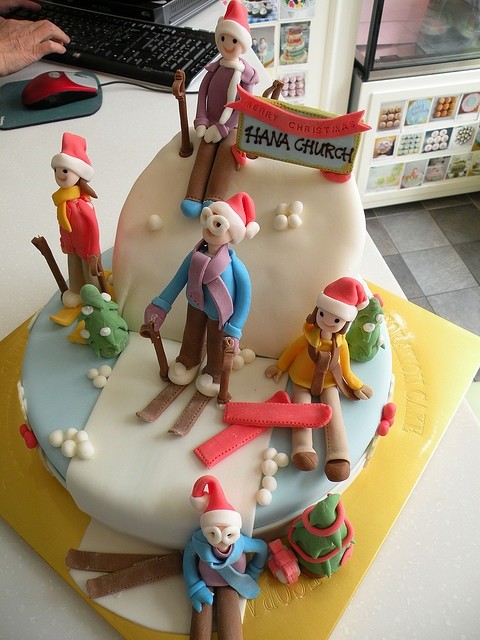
[{"xmin": 17, "ymin": 1, "xmax": 392, "ymax": 640}]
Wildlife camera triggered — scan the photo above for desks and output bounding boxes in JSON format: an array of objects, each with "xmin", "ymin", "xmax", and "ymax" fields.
[{"xmin": 0, "ymin": 0, "xmax": 477, "ymax": 639}]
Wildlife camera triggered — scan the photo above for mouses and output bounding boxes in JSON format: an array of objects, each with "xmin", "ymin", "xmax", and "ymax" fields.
[{"xmin": 21, "ymin": 69, "xmax": 101, "ymax": 110}]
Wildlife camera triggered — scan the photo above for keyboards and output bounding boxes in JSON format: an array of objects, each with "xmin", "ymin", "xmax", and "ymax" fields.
[{"xmin": 3, "ymin": 0, "xmax": 220, "ymax": 94}]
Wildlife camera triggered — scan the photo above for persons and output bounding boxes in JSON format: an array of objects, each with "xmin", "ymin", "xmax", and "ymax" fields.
[
  {"xmin": 1, "ymin": 0, "xmax": 70, "ymax": 77},
  {"xmin": 251, "ymin": 38, "xmax": 260, "ymax": 60},
  {"xmin": 258, "ymin": 38, "xmax": 268, "ymax": 64}
]
